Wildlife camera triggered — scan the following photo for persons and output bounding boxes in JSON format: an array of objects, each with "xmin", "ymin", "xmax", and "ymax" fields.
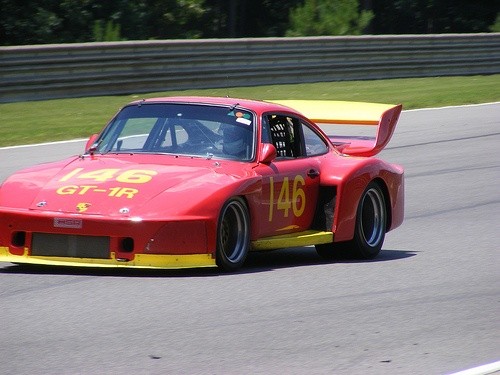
[{"xmin": 207, "ymin": 121, "xmax": 250, "ymax": 159}]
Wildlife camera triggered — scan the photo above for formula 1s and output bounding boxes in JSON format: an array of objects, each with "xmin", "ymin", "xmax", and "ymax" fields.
[{"xmin": 2, "ymin": 96, "xmax": 406, "ymax": 273}]
